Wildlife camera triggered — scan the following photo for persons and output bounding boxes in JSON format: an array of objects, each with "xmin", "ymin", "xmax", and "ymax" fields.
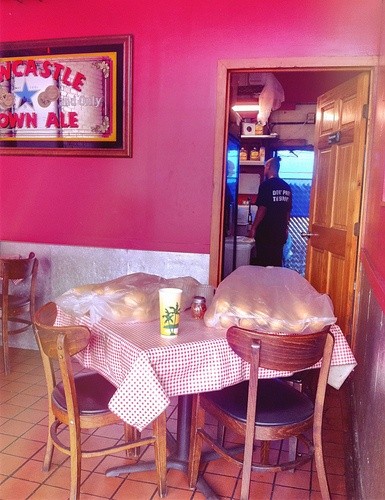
[{"xmin": 248, "ymin": 157, "xmax": 292, "ymax": 267}]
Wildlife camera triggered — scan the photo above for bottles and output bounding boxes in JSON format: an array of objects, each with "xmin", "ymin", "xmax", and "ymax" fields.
[
  {"xmin": 250, "ymin": 147, "xmax": 259, "ymax": 161},
  {"xmin": 255, "ymin": 121, "xmax": 263, "ymax": 136},
  {"xmin": 248, "ymin": 212, "xmax": 252, "ymax": 224},
  {"xmin": 239, "ymin": 147, "xmax": 247, "ymax": 162},
  {"xmin": 191, "ymin": 296, "xmax": 207, "ymax": 320}
]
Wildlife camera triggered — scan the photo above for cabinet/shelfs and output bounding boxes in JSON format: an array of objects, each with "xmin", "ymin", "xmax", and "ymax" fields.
[{"xmin": 238, "ymin": 132, "xmax": 279, "ymax": 166}]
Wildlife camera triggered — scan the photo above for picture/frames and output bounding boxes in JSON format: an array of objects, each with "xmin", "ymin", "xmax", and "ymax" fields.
[{"xmin": 1, "ymin": 32, "xmax": 133, "ymax": 159}]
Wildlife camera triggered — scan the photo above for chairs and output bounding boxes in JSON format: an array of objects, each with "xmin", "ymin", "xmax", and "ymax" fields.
[
  {"xmin": 0, "ymin": 252, "xmax": 39, "ymax": 376},
  {"xmin": 187, "ymin": 324, "xmax": 336, "ymax": 499},
  {"xmin": 33, "ymin": 301, "xmax": 168, "ymax": 500}
]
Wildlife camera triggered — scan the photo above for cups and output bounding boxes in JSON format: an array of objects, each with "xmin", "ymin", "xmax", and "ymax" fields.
[
  {"xmin": 196, "ymin": 284, "xmax": 215, "ymax": 308},
  {"xmin": 158, "ymin": 288, "xmax": 183, "ymax": 339}
]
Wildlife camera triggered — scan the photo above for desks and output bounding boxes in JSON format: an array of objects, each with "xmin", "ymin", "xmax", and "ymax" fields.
[{"xmin": 53, "ymin": 300, "xmax": 357, "ymax": 500}]
